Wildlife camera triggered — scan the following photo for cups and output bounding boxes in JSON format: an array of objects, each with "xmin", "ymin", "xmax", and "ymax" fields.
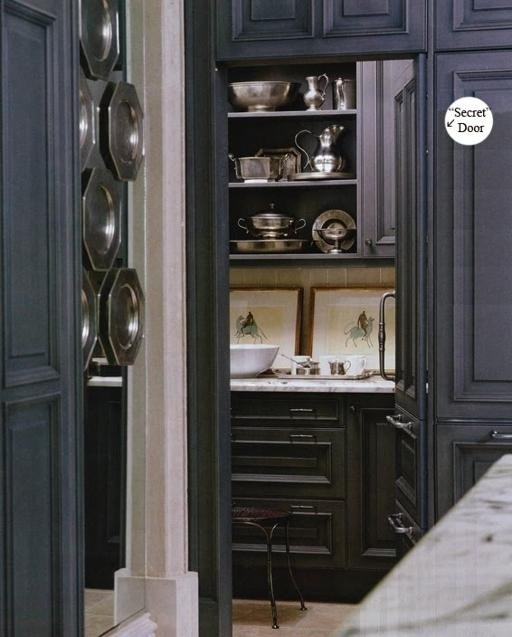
[
  {"xmin": 319, "ymin": 355, "xmax": 337, "ymax": 376},
  {"xmin": 291, "ymin": 356, "xmax": 313, "ymax": 375},
  {"xmin": 345, "ymin": 356, "xmax": 367, "ymax": 375},
  {"xmin": 333, "ymin": 77, "xmax": 356, "ymax": 110}
]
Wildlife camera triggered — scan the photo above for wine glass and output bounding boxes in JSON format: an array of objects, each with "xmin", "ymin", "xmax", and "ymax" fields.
[{"xmin": 314, "ymin": 229, "xmax": 357, "ymax": 253}]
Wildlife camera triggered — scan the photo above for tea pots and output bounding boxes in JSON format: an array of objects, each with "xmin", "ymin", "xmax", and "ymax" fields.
[
  {"xmin": 326, "ymin": 360, "xmax": 351, "ymax": 375},
  {"xmin": 293, "ymin": 122, "xmax": 346, "ymax": 173}
]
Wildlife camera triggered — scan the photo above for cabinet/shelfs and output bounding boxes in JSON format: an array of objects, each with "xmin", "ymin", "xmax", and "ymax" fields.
[
  {"xmin": 222, "ymin": 0, "xmax": 427, "ymax": 262},
  {"xmin": 85, "ymin": 384, "xmax": 396, "ymax": 604},
  {"xmin": 384, "ymin": 50, "xmax": 512, "ymax": 567}
]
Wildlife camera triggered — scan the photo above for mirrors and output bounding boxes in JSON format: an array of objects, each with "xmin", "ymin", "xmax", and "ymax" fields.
[{"xmin": 87, "ymin": 359, "xmax": 148, "ymax": 637}]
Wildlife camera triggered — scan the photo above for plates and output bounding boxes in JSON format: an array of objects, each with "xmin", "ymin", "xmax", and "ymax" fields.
[{"xmin": 312, "ymin": 209, "xmax": 356, "ymax": 253}]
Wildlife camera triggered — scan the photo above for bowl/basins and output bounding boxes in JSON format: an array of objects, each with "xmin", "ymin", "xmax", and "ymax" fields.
[
  {"xmin": 226, "ymin": 80, "xmax": 302, "ymax": 111},
  {"xmin": 230, "ymin": 344, "xmax": 280, "ymax": 380}
]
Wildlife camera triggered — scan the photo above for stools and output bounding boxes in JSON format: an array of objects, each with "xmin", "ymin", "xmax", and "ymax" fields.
[{"xmin": 232, "ymin": 498, "xmax": 307, "ymax": 630}]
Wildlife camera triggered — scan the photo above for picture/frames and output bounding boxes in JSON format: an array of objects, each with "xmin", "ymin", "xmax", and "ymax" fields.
[
  {"xmin": 228, "ymin": 284, "xmax": 305, "ymax": 376},
  {"xmin": 305, "ymin": 285, "xmax": 396, "ymax": 376}
]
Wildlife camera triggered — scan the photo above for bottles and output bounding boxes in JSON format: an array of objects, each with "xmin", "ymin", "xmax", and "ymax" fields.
[{"xmin": 303, "ymin": 72, "xmax": 329, "ymax": 111}]
